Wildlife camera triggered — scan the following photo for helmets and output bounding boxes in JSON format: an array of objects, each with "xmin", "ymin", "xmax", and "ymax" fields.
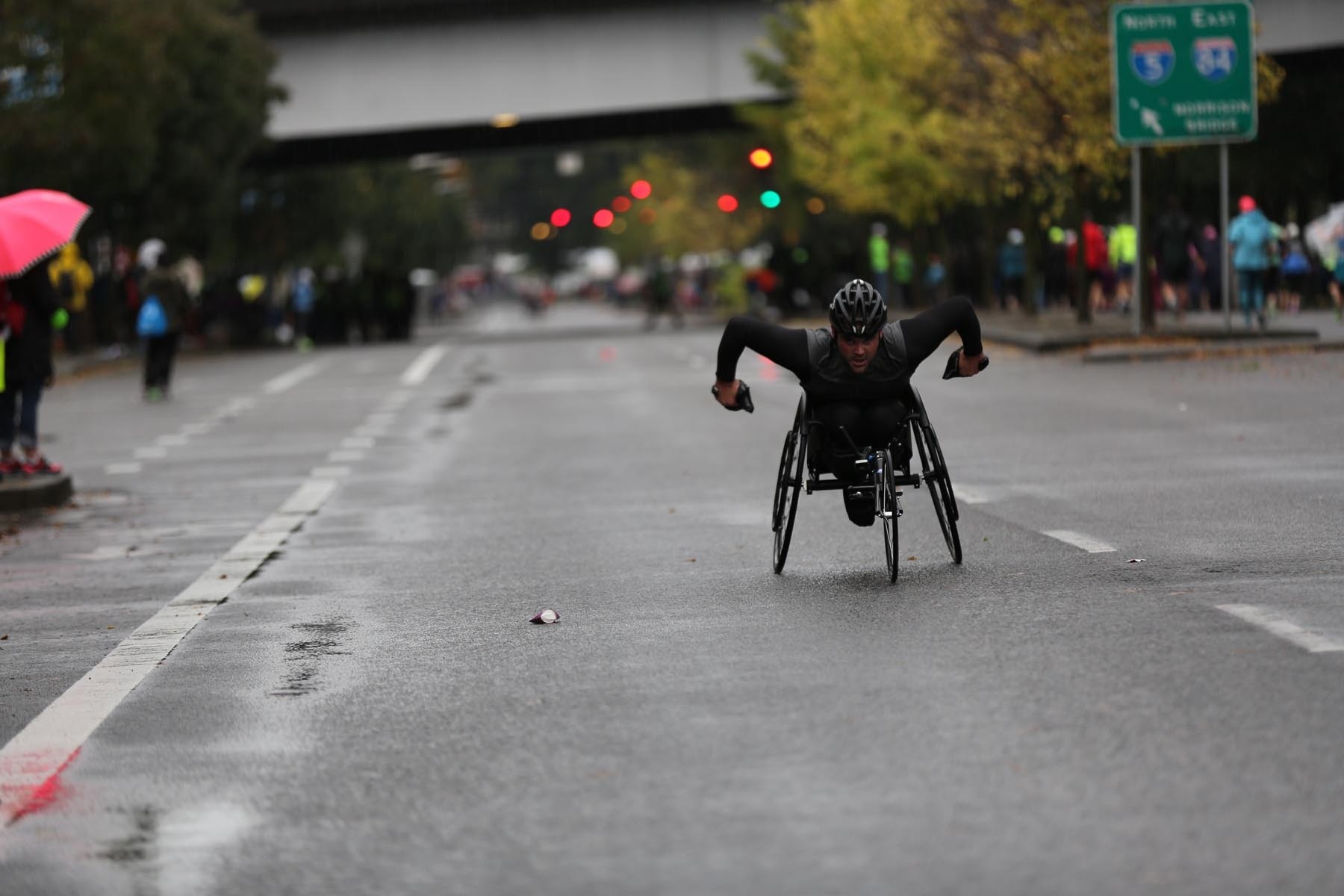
[{"xmin": 829, "ymin": 279, "xmax": 888, "ymax": 339}]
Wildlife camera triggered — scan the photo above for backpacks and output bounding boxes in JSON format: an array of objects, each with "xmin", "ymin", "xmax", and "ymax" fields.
[{"xmin": 137, "ymin": 294, "xmax": 166, "ymax": 337}]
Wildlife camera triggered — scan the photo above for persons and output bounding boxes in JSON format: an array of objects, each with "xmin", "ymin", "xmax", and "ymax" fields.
[
  {"xmin": 717, "ymin": 278, "xmax": 985, "ymax": 527},
  {"xmin": 1, "ymin": 195, "xmax": 1343, "ymax": 480}
]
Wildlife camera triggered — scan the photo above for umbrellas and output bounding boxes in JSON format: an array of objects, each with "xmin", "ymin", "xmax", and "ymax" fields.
[{"xmin": 1, "ymin": 187, "xmax": 94, "ymax": 282}]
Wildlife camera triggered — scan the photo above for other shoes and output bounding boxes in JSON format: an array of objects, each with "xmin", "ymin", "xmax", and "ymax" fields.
[
  {"xmin": 0, "ymin": 459, "xmax": 21, "ymax": 473},
  {"xmin": 23, "ymin": 457, "xmax": 60, "ymax": 474}
]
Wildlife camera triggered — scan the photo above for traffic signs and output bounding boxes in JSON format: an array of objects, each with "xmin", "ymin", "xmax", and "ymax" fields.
[{"xmin": 1110, "ymin": 1, "xmax": 1260, "ymax": 147}]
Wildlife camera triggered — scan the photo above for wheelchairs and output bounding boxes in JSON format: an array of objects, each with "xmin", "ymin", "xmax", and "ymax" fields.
[{"xmin": 770, "ymin": 382, "xmax": 963, "ymax": 585}]
